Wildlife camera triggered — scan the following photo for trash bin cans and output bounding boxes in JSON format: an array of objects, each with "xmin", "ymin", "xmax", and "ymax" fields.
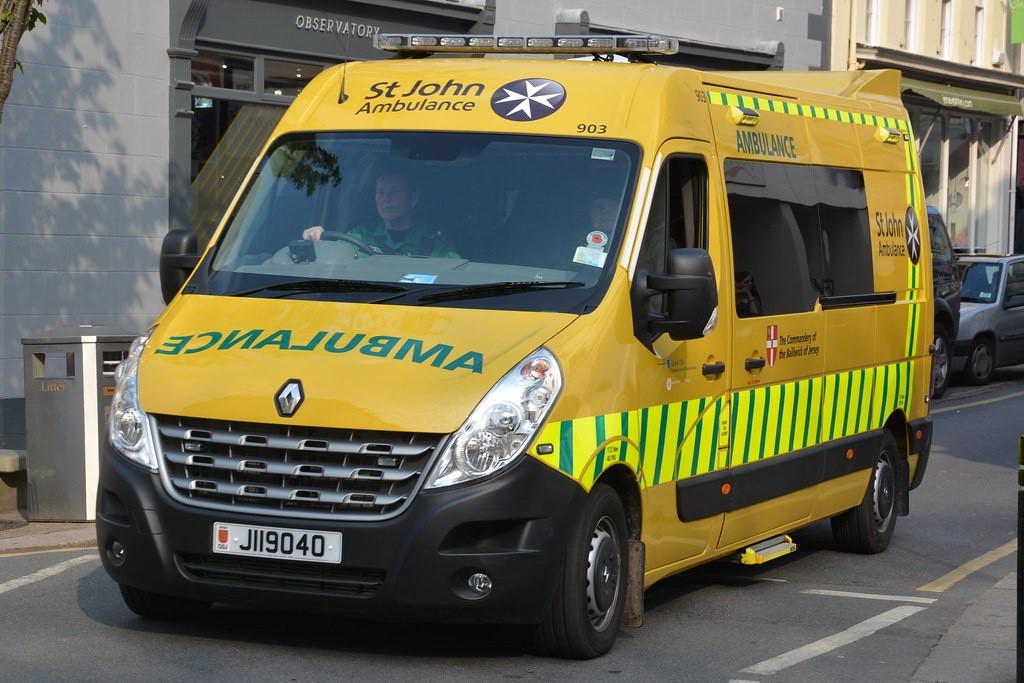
[{"xmin": 20, "ymin": 324, "xmax": 144, "ymax": 524}]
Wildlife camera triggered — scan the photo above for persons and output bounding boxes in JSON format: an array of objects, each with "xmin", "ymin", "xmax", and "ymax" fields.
[
  {"xmin": 590, "ymin": 190, "xmax": 677, "ymax": 313},
  {"xmin": 302, "ymin": 167, "xmax": 460, "ymax": 258}
]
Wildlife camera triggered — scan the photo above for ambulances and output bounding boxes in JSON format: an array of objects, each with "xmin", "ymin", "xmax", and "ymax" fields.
[{"xmin": 98, "ymin": 31, "xmax": 935, "ymax": 661}]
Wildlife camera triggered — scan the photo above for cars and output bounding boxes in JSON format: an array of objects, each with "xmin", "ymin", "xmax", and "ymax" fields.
[
  {"xmin": 928, "ymin": 206, "xmax": 960, "ymax": 398},
  {"xmin": 953, "ymin": 253, "xmax": 1024, "ymax": 387}
]
